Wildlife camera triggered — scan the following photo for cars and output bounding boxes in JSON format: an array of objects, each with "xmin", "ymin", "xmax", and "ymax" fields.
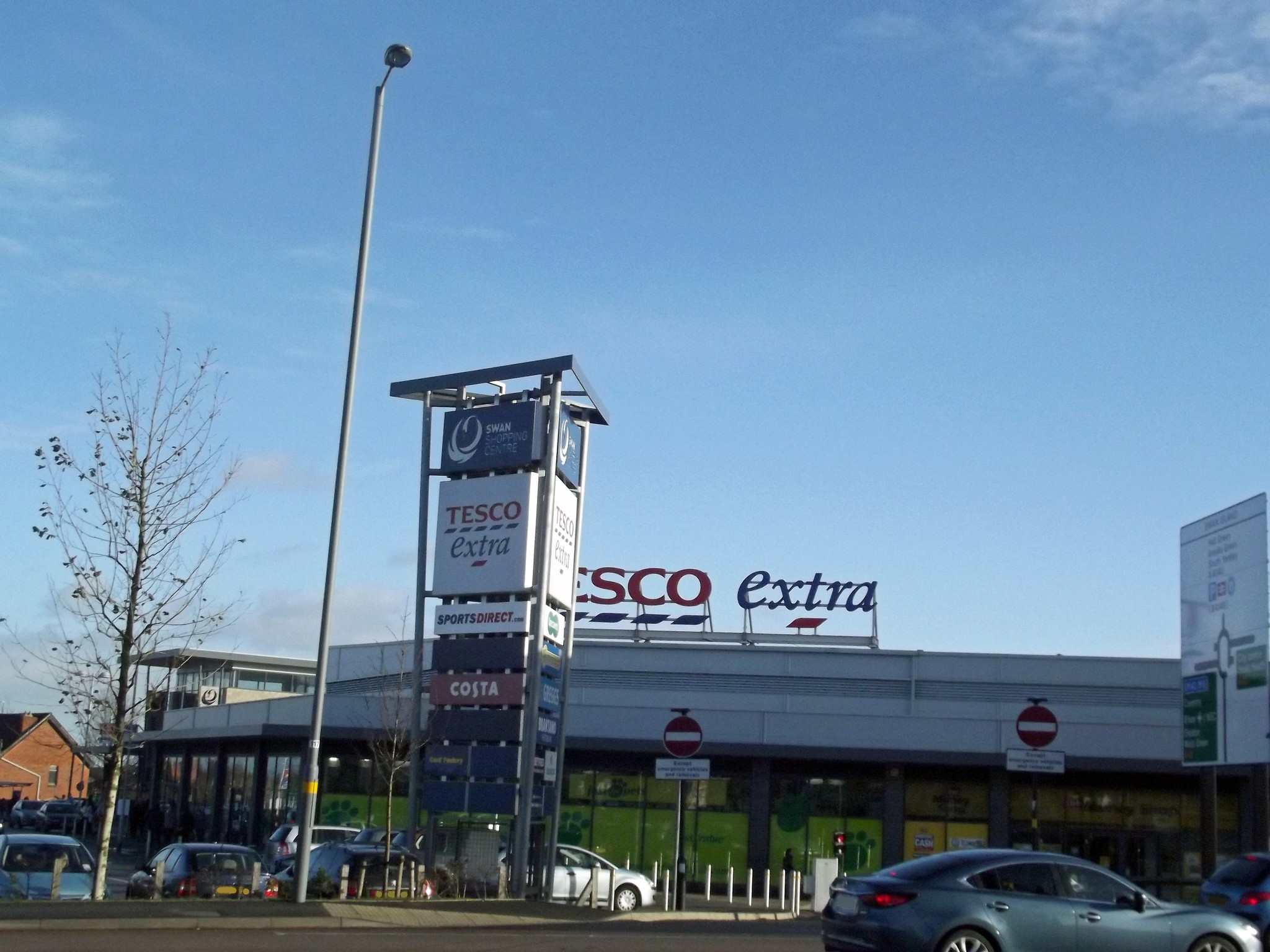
[
  {"xmin": 1197, "ymin": 852, "xmax": 1270, "ymax": 932},
  {"xmin": 0, "ymin": 794, "xmax": 98, "ymax": 833},
  {"xmin": 262, "ymin": 823, "xmax": 504, "ymax": 906},
  {"xmin": 818, "ymin": 847, "xmax": 1263, "ymax": 952},
  {"xmin": 497, "ymin": 842, "xmax": 657, "ymax": 912},
  {"xmin": 0, "ymin": 833, "xmax": 111, "ymax": 901},
  {"xmin": 126, "ymin": 841, "xmax": 267, "ymax": 901}
]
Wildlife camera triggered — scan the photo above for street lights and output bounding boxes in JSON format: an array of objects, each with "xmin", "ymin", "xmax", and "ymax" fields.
[{"xmin": 299, "ymin": 38, "xmax": 412, "ymax": 907}]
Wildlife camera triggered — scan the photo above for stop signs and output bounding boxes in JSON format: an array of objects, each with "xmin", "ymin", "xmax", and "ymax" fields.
[
  {"xmin": 1016, "ymin": 705, "xmax": 1058, "ymax": 748},
  {"xmin": 662, "ymin": 716, "xmax": 703, "ymax": 757}
]
